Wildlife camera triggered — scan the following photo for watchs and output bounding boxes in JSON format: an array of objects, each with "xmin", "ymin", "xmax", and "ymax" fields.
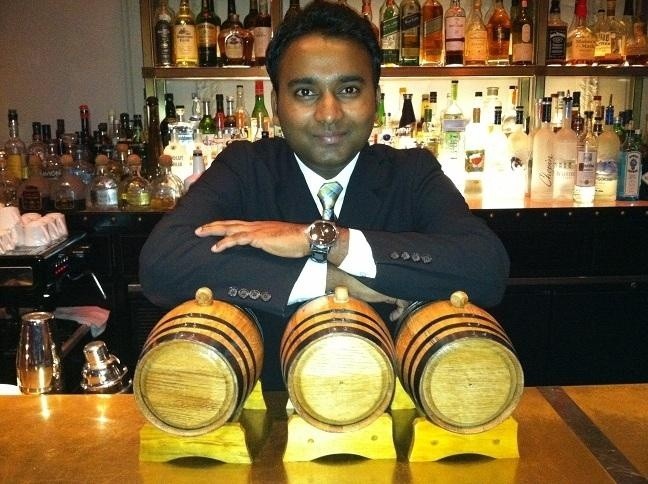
[{"xmin": 303, "ymin": 220, "xmax": 339, "ymax": 264}]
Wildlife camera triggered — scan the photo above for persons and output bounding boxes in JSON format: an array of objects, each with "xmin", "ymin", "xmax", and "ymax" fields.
[{"xmin": 137, "ymin": 1, "xmax": 511, "ymax": 392}]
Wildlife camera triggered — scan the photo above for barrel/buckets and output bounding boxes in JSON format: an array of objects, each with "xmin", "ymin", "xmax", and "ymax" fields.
[
  {"xmin": 393, "ymin": 290, "xmax": 525, "ymax": 435},
  {"xmin": 279, "ymin": 287, "xmax": 398, "ymax": 435},
  {"xmin": 132, "ymin": 289, "xmax": 265, "ymax": 438}
]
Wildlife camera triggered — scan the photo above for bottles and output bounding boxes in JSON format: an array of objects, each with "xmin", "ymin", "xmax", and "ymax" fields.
[
  {"xmin": 79, "ymin": 342, "xmax": 132, "ymax": 395},
  {"xmin": 151, "ymin": 0, "xmax": 299, "ymax": 70},
  {"xmin": 1, "ymin": 78, "xmax": 277, "ymax": 209},
  {"xmin": 368, "ymin": 81, "xmax": 648, "ymax": 202},
  {"xmin": 343, "ymin": 0, "xmax": 644, "ymax": 67}
]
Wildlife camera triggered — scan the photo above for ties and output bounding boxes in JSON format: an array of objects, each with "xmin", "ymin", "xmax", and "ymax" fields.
[{"xmin": 316, "ymin": 181, "xmax": 344, "ymax": 221}]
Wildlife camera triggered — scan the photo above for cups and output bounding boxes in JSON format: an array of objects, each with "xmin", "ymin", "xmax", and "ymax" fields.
[{"xmin": 17, "ymin": 312, "xmax": 65, "ymax": 393}]
